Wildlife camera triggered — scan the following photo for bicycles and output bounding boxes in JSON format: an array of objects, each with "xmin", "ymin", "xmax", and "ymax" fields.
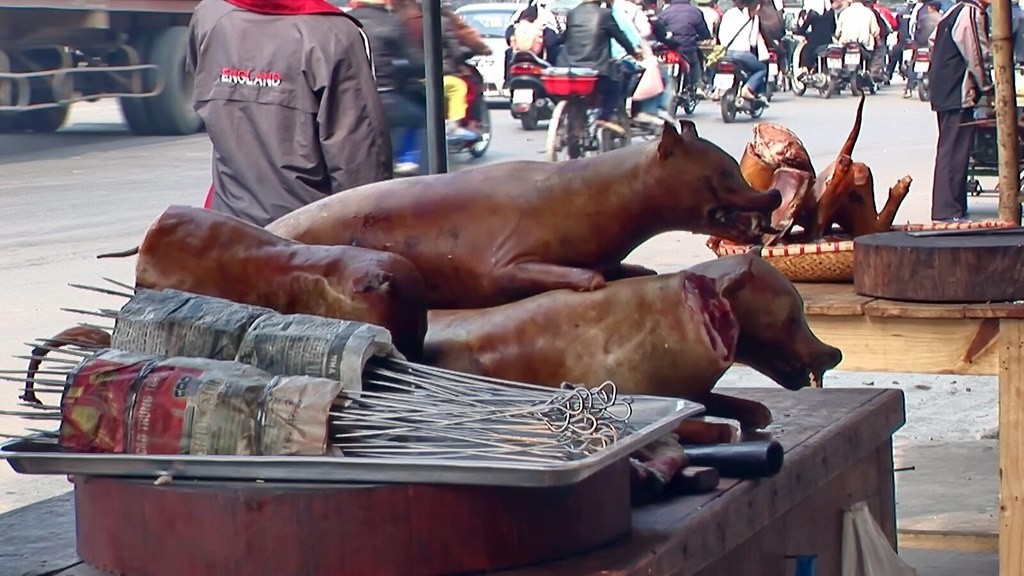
[{"xmin": 537, "ymin": 53, "xmax": 665, "ymax": 163}]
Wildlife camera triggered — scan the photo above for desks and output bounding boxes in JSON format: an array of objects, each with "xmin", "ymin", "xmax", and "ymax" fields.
[
  {"xmin": 791, "ymin": 279, "xmax": 1024, "ymax": 576},
  {"xmin": 1, "ymin": 389, "xmax": 904, "ymax": 576}
]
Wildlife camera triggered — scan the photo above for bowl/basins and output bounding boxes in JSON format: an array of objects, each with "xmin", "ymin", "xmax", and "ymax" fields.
[{"xmin": 540, "ymin": 76, "xmax": 599, "ymax": 96}]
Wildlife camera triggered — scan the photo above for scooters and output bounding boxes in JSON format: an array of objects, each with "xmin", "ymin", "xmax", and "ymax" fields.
[{"xmin": 502, "ymin": 52, "xmax": 566, "ymax": 131}]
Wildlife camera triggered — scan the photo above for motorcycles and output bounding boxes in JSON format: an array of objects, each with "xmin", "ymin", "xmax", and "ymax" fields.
[
  {"xmin": 390, "ymin": 49, "xmax": 493, "ymax": 178},
  {"xmin": 652, "ymin": 0, "xmax": 935, "ymax": 124}
]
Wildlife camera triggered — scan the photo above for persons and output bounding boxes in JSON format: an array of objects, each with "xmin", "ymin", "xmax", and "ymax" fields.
[
  {"xmin": 184, "ymin": 0, "xmax": 395, "ymax": 227},
  {"xmin": 503, "ymin": 0, "xmax": 792, "ymax": 124},
  {"xmin": 345, "ymin": 1, "xmax": 491, "ymax": 176},
  {"xmin": 792, "ymin": 0, "xmax": 1024, "ymax": 98},
  {"xmin": 928, "ymin": 0, "xmax": 995, "ymax": 224}
]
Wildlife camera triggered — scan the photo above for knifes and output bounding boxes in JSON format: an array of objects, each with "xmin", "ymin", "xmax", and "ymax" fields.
[{"xmin": 906, "ymin": 225, "xmax": 1024, "ymax": 238}]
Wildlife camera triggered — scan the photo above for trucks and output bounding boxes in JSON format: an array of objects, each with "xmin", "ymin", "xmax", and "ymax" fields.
[{"xmin": 0, "ymin": 0, "xmax": 206, "ymax": 138}]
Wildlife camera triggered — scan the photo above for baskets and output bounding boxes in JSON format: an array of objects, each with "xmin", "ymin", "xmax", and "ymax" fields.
[{"xmin": 716, "ymin": 221, "xmax": 1016, "ymax": 281}]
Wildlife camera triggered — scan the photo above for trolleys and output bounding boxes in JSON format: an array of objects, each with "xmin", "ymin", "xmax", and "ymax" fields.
[{"xmin": 963, "ymin": 64, "xmax": 1024, "ymax": 196}]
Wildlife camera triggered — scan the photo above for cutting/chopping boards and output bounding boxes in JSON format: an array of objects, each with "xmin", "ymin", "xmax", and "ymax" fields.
[
  {"xmin": 853, "ymin": 231, "xmax": 1024, "ymax": 303},
  {"xmin": 68, "ymin": 454, "xmax": 632, "ymax": 576}
]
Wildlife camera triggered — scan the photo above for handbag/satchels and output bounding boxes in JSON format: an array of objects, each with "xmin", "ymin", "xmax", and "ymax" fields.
[
  {"xmin": 630, "ymin": 57, "xmax": 665, "ymax": 101},
  {"xmin": 706, "ymin": 44, "xmax": 727, "ymax": 68}
]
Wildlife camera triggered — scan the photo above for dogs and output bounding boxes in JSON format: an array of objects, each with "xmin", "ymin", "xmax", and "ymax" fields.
[{"xmin": 102, "ymin": 88, "xmax": 913, "ymax": 487}]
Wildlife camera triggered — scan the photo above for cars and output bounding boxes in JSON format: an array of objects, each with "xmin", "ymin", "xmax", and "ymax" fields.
[{"xmin": 452, "ymin": 2, "xmax": 529, "ymax": 109}]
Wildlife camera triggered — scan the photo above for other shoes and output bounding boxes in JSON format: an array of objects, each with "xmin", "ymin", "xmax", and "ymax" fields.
[
  {"xmin": 741, "ymin": 87, "xmax": 758, "ymax": 100},
  {"xmin": 871, "ymin": 74, "xmax": 885, "ymax": 82},
  {"xmin": 446, "ymin": 127, "xmax": 478, "ymax": 141},
  {"xmin": 633, "ymin": 113, "xmax": 665, "ymax": 125},
  {"xmin": 933, "ymin": 217, "xmax": 973, "ymax": 224},
  {"xmin": 393, "ymin": 161, "xmax": 420, "ymax": 173},
  {"xmin": 883, "ymin": 80, "xmax": 890, "ymax": 85},
  {"xmin": 904, "ymin": 91, "xmax": 912, "ymax": 98},
  {"xmin": 757, "ymin": 94, "xmax": 769, "ymax": 107}
]
